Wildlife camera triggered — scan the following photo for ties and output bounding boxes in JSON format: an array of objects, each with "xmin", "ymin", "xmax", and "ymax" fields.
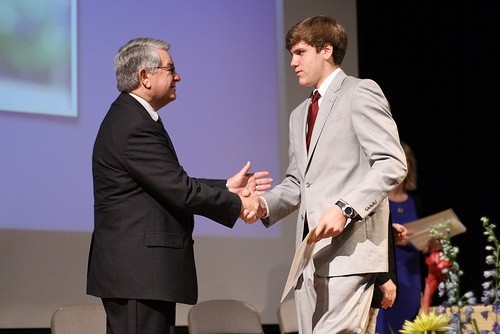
[{"xmin": 307, "ymin": 91, "xmax": 322, "ymax": 153}]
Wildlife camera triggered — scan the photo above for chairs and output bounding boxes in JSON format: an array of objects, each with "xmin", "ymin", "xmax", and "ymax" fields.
[
  {"xmin": 51, "ymin": 303, "xmax": 107, "ymax": 334},
  {"xmin": 277, "ymin": 298, "xmax": 300, "ymax": 334},
  {"xmin": 186, "ymin": 298, "xmax": 266, "ymax": 334}
]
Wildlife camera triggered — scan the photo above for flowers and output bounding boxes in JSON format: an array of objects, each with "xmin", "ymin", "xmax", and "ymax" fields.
[{"xmin": 398, "ymin": 217, "xmax": 500, "ymax": 334}]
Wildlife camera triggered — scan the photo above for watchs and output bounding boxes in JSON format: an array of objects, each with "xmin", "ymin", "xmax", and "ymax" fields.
[{"xmin": 335, "ymin": 200, "xmax": 355, "ymax": 222}]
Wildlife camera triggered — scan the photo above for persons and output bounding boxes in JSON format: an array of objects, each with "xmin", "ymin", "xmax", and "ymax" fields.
[
  {"xmin": 87, "ymin": 37, "xmax": 274, "ymax": 334},
  {"xmin": 241, "ymin": 16, "xmax": 409, "ymax": 333},
  {"xmin": 361, "ymin": 145, "xmax": 443, "ymax": 334}
]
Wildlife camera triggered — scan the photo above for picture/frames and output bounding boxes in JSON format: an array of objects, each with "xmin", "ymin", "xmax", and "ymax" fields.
[{"xmin": 0, "ymin": 0, "xmax": 80, "ymax": 119}]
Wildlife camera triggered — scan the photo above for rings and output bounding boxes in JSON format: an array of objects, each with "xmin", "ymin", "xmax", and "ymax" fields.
[{"xmin": 255, "ymin": 185, "xmax": 259, "ymax": 191}]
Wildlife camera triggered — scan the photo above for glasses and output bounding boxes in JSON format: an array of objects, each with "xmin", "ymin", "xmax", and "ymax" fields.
[{"xmin": 155, "ymin": 65, "xmax": 176, "ymax": 75}]
